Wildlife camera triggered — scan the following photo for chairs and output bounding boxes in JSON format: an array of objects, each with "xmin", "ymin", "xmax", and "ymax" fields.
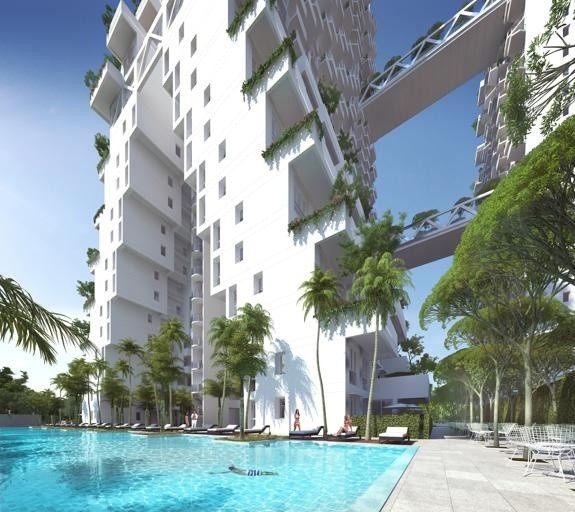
[
  {"xmin": 331, "ymin": 425, "xmax": 361, "ymax": 440},
  {"xmin": 378, "ymin": 426, "xmax": 410, "ymax": 444},
  {"xmin": 234, "ymin": 424, "xmax": 270, "ymax": 435},
  {"xmin": 206, "ymin": 424, "xmax": 239, "ymax": 435},
  {"xmin": 289, "ymin": 425, "xmax": 324, "ymax": 439},
  {"xmin": 44, "ymin": 421, "xmax": 218, "ymax": 433},
  {"xmin": 447, "ymin": 421, "xmax": 575, "ymax": 485}
]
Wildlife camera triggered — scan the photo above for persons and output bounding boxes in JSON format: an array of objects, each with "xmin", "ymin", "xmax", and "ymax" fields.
[
  {"xmin": 294, "ymin": 409, "xmax": 301, "ymax": 431},
  {"xmin": 208, "ymin": 463, "xmax": 279, "ymax": 476},
  {"xmin": 334, "ymin": 414, "xmax": 352, "ymax": 437},
  {"xmin": 184, "ymin": 410, "xmax": 198, "ymax": 428}
]
